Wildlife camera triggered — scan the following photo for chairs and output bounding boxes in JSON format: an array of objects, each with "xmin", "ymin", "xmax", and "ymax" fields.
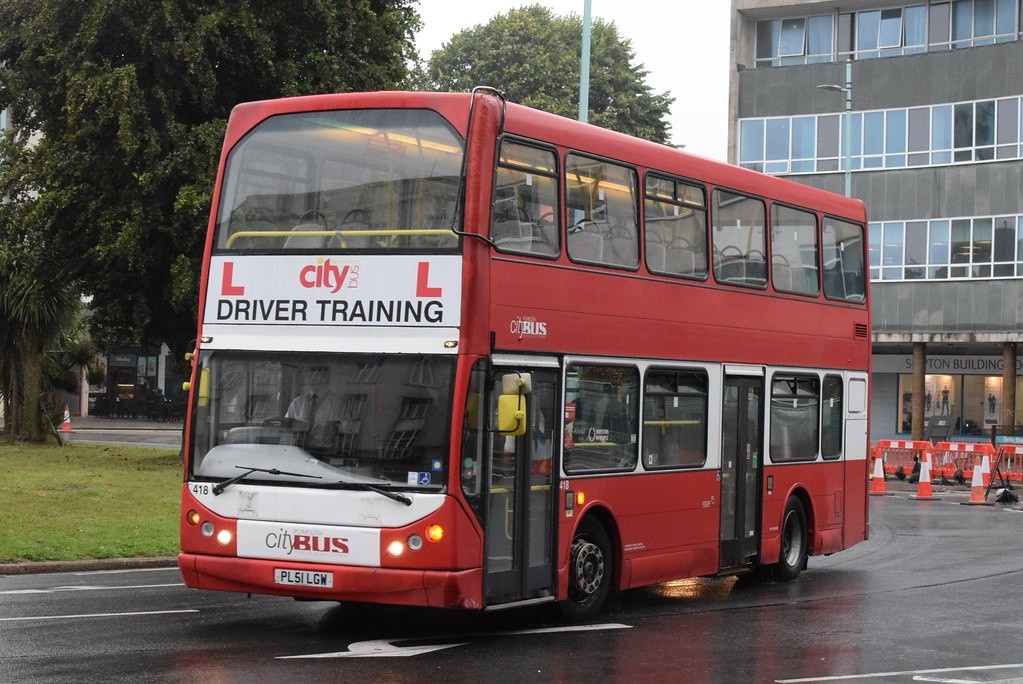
[{"xmin": 226, "ymin": 206, "xmax": 863, "ymax": 300}]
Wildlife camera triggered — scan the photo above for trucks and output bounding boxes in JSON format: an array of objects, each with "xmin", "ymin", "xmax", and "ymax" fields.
[{"xmin": 117, "ymin": 384, "xmax": 135, "ymax": 401}]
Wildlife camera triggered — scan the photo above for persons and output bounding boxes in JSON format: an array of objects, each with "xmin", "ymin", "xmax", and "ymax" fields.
[
  {"xmin": 941, "ymin": 384, "xmax": 950, "ymax": 416},
  {"xmin": 906, "ymin": 397, "xmax": 912, "ymax": 426},
  {"xmin": 273, "ymin": 366, "xmax": 352, "ymax": 449},
  {"xmin": 594, "ymin": 383, "xmax": 630, "ymax": 443},
  {"xmin": 988, "ymin": 393, "xmax": 996, "ymax": 413},
  {"xmin": 926, "ymin": 391, "xmax": 932, "ymax": 411}
]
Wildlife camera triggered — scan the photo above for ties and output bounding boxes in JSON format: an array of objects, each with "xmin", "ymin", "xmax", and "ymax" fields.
[{"xmin": 308, "ymin": 394, "xmax": 317, "ymax": 430}]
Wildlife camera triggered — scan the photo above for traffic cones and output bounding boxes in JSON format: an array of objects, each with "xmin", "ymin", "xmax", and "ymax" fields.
[
  {"xmin": 907, "ymin": 451, "xmax": 942, "ymax": 502},
  {"xmin": 960, "ymin": 446, "xmax": 994, "ymax": 506},
  {"xmin": 58, "ymin": 403, "xmax": 75, "ymax": 433},
  {"xmin": 869, "ymin": 450, "xmax": 896, "ymax": 497}
]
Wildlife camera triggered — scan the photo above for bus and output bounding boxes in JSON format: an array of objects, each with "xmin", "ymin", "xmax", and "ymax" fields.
[
  {"xmin": 88, "ymin": 391, "xmax": 106, "ymax": 408},
  {"xmin": 177, "ymin": 85, "xmax": 872, "ymax": 634}
]
